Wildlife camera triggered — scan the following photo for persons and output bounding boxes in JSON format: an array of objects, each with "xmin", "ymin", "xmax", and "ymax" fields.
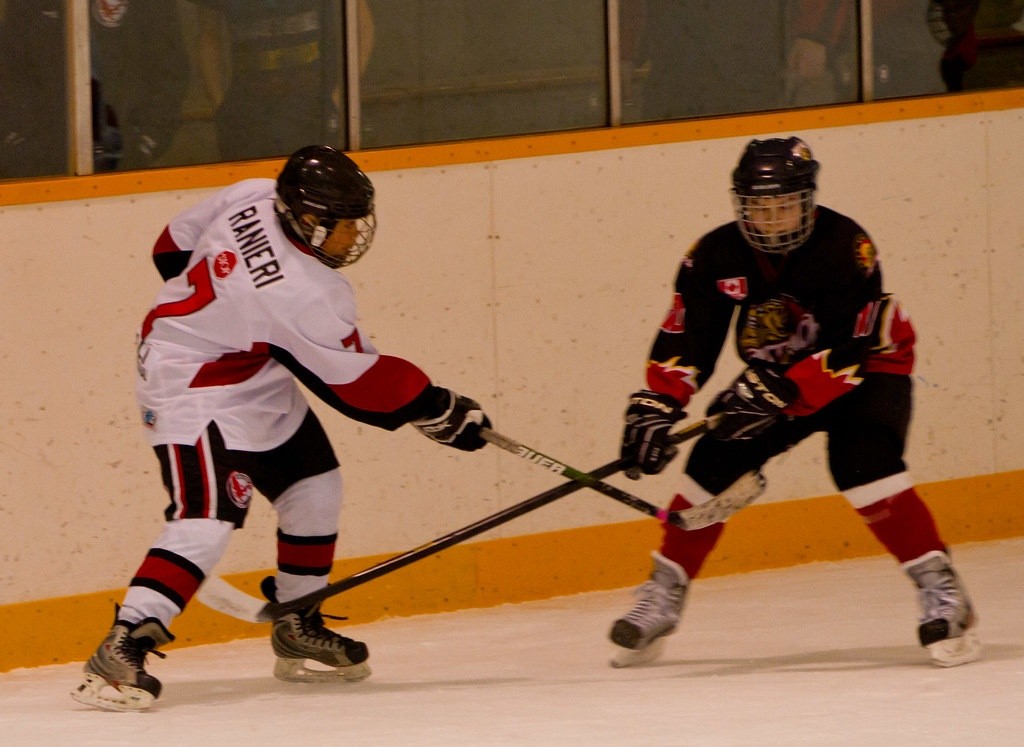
[
  {"xmin": 610, "ymin": 0, "xmax": 859, "ymax": 119},
  {"xmin": 1, "ymin": 0, "xmax": 378, "ymax": 180},
  {"xmin": 72, "ymin": 144, "xmax": 491, "ymax": 715},
  {"xmin": 924, "ymin": 1, "xmax": 1024, "ymax": 93},
  {"xmin": 599, "ymin": 136, "xmax": 980, "ymax": 667}
]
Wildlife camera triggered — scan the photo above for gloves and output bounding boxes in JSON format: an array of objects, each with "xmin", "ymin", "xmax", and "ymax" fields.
[
  {"xmin": 706, "ymin": 363, "xmax": 800, "ymax": 442},
  {"xmin": 410, "ymin": 386, "xmax": 491, "ymax": 452},
  {"xmin": 620, "ymin": 391, "xmax": 688, "ymax": 478}
]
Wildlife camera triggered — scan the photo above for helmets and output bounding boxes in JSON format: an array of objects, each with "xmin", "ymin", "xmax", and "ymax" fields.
[
  {"xmin": 729, "ymin": 136, "xmax": 820, "ymax": 253},
  {"xmin": 277, "ymin": 144, "xmax": 378, "ymax": 269}
]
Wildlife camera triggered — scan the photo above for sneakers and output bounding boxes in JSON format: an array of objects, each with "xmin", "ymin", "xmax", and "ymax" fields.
[
  {"xmin": 900, "ymin": 550, "xmax": 987, "ymax": 665},
  {"xmin": 608, "ymin": 550, "xmax": 692, "ymax": 668},
  {"xmin": 261, "ymin": 575, "xmax": 371, "ymax": 684},
  {"xmin": 71, "ymin": 602, "xmax": 177, "ymax": 712}
]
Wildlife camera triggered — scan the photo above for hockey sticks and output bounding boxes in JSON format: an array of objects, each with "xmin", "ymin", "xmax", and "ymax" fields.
[{"xmin": 193, "ymin": 413, "xmax": 722, "ymax": 625}]
